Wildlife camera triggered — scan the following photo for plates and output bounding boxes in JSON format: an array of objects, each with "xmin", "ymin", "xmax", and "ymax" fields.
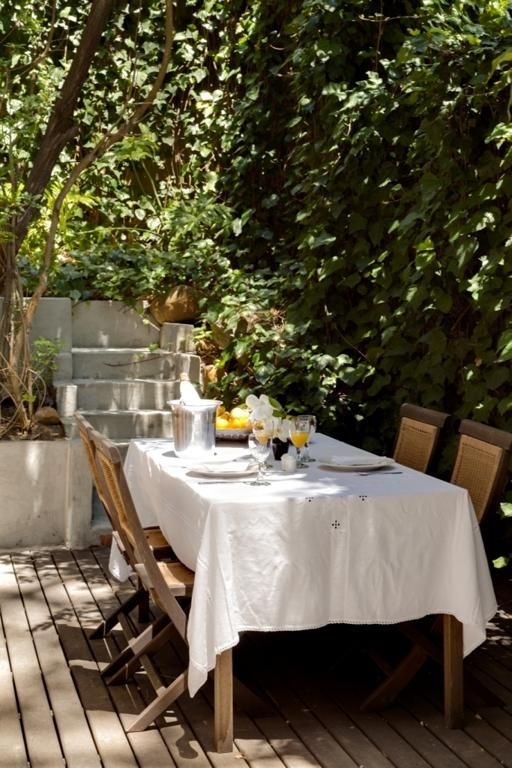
[
  {"xmin": 318, "ymin": 452, "xmax": 394, "ymax": 471},
  {"xmin": 184, "ymin": 463, "xmax": 262, "ymax": 478}
]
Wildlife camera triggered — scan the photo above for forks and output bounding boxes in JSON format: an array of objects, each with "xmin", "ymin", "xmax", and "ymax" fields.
[{"xmin": 197, "ymin": 479, "xmax": 272, "ymax": 488}]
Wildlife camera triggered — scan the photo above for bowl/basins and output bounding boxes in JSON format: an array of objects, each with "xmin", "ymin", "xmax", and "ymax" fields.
[{"xmin": 213, "ymin": 427, "xmax": 251, "ymax": 440}]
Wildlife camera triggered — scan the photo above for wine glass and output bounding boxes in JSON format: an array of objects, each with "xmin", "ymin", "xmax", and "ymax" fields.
[{"xmin": 248, "ymin": 413, "xmax": 317, "ymax": 487}]
